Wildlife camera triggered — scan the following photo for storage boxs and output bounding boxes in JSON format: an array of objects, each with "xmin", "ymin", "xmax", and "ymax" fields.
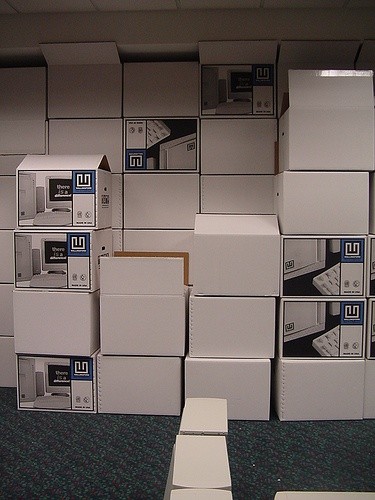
[{"xmin": 0, "ymin": 38, "xmax": 375, "ymax": 500}]
